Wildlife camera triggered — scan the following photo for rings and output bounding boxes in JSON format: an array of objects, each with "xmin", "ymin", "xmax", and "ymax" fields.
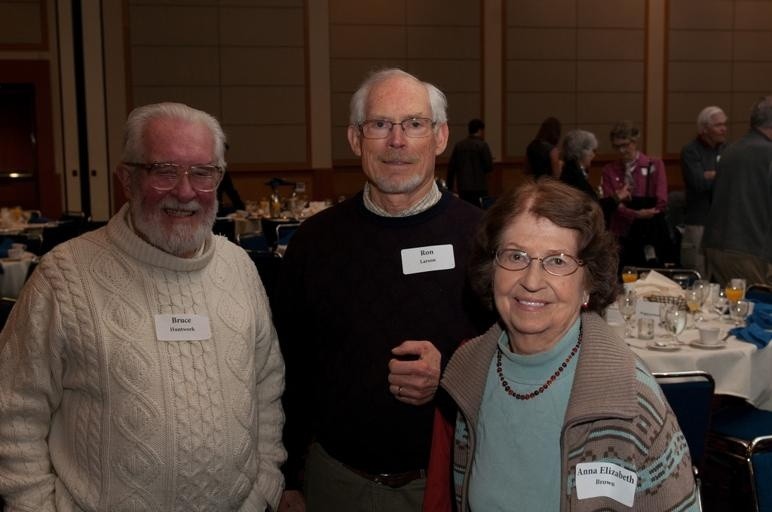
[{"xmin": 398, "ymin": 386, "xmax": 403, "ymax": 397}]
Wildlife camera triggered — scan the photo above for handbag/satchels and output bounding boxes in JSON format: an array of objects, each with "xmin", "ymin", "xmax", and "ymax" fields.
[{"xmin": 627, "ymin": 157, "xmax": 674, "ymax": 243}]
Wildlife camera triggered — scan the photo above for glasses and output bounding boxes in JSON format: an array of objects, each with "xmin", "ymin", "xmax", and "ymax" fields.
[
  {"xmin": 126, "ymin": 162, "xmax": 223, "ymax": 192},
  {"xmin": 357, "ymin": 116, "xmax": 436, "ymax": 139},
  {"xmin": 492, "ymin": 249, "xmax": 584, "ymax": 277}
]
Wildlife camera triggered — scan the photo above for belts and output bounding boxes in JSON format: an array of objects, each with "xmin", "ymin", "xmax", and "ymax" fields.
[{"xmin": 344, "ymin": 465, "xmax": 426, "ymax": 488}]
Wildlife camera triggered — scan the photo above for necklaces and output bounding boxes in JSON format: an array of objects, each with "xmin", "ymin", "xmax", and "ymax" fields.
[{"xmin": 496, "ymin": 327, "xmax": 583, "ymax": 400}]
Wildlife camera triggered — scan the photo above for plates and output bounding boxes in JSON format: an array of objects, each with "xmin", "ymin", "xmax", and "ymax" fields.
[{"xmin": 648, "ymin": 342, "xmax": 731, "ymax": 352}]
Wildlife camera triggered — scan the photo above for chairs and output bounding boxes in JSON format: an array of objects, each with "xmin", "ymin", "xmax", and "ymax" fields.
[
  {"xmin": 625, "ymin": 266, "xmax": 702, "ymax": 286},
  {"xmin": 214, "ymin": 193, "xmax": 335, "ymax": 289},
  {"xmin": 747, "ymin": 435, "xmax": 772, "ymax": 512},
  {"xmin": 746, "ymin": 284, "xmax": 772, "ymax": 304},
  {"xmin": 26, "ymin": 313, "xmax": 32, "ymax": 318},
  {"xmin": 718, "ymin": 413, "xmax": 772, "ymax": 462},
  {"xmin": 653, "ymin": 371, "xmax": 715, "ymax": 469},
  {"xmin": 700, "ymin": 437, "xmax": 763, "ymax": 512},
  {"xmin": 0, "ymin": 207, "xmax": 109, "ymax": 332}
]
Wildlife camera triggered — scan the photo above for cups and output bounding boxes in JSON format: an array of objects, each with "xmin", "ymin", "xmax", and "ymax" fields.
[{"xmin": 699, "ymin": 327, "xmax": 723, "ymax": 345}]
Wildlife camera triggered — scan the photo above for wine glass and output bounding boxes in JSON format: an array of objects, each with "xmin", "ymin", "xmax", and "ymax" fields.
[
  {"xmin": 0, "ymin": 206, "xmax": 30, "ymax": 271},
  {"xmin": 240, "ymin": 190, "xmax": 322, "ymax": 220},
  {"xmin": 615, "ymin": 266, "xmax": 750, "ymax": 339}
]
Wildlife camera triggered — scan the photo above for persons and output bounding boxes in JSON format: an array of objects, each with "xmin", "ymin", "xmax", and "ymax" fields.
[
  {"xmin": 269, "ymin": 66, "xmax": 499, "ymax": 512},
  {"xmin": 602, "ymin": 119, "xmax": 669, "ymax": 268},
  {"xmin": 674, "ymin": 104, "xmax": 728, "ymax": 283},
  {"xmin": 560, "ymin": 129, "xmax": 633, "ymax": 230},
  {"xmin": 420, "ymin": 173, "xmax": 702, "ymax": 512},
  {"xmin": 524, "ymin": 117, "xmax": 564, "ymax": 181},
  {"xmin": 0, "ymin": 101, "xmax": 290, "ymax": 512},
  {"xmin": 217, "ymin": 142, "xmax": 245, "ymax": 217},
  {"xmin": 703, "ymin": 94, "xmax": 772, "ymax": 289},
  {"xmin": 446, "ymin": 118, "xmax": 493, "ymax": 209}
]
